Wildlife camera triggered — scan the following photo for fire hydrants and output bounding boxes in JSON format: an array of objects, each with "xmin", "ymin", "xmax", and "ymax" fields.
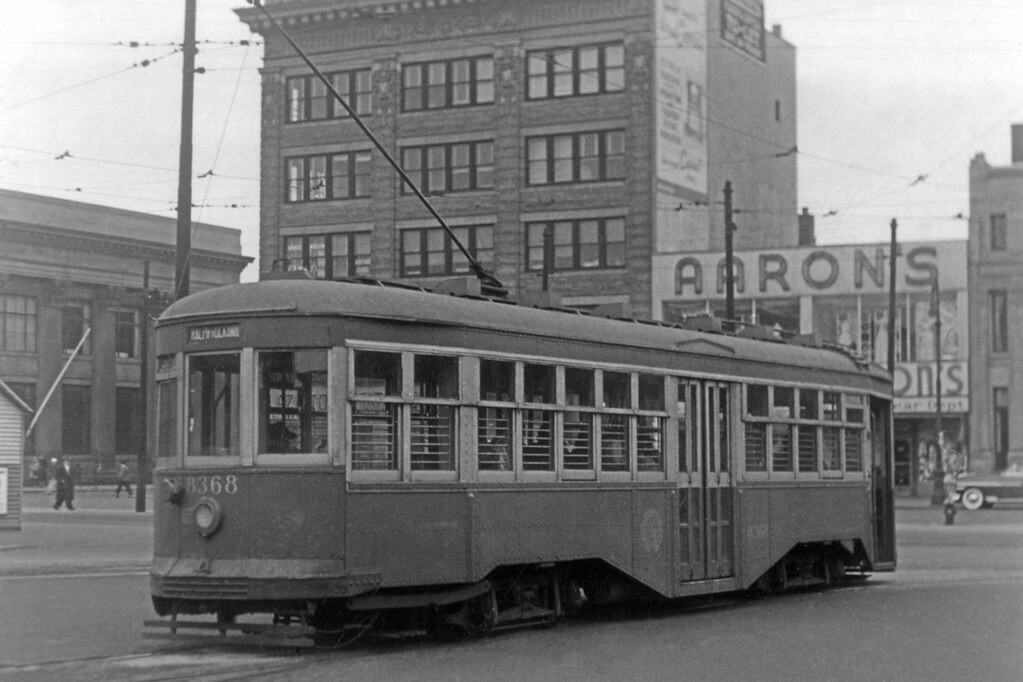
[{"xmin": 943, "ymin": 499, "xmax": 957, "ymax": 525}]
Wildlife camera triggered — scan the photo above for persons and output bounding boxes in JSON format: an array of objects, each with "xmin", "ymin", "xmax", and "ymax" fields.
[
  {"xmin": 54, "ymin": 460, "xmax": 75, "ymax": 510},
  {"xmin": 115, "ymin": 461, "xmax": 133, "ymax": 498},
  {"xmin": 48, "ymin": 458, "xmax": 57, "ymax": 493}
]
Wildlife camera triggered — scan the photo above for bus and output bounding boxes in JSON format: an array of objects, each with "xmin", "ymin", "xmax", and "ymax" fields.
[{"xmin": 150, "ymin": 274, "xmax": 894, "ymax": 649}]
